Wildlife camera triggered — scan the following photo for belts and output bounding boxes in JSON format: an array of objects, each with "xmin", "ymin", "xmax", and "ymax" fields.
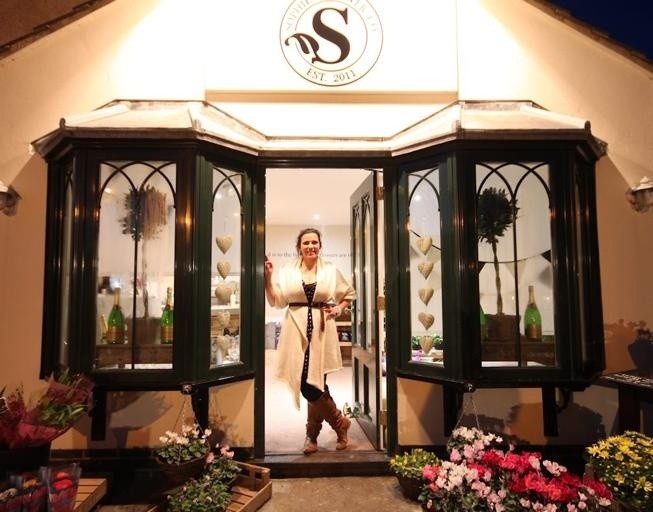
[{"xmin": 289, "ymin": 302, "xmax": 329, "ymax": 331}]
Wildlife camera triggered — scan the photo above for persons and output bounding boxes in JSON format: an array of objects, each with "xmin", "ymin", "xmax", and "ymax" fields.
[{"xmin": 265, "ymin": 228, "xmax": 357, "ymax": 454}]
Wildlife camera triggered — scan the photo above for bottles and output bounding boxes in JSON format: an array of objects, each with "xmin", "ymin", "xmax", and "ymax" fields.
[
  {"xmin": 524, "ymin": 286, "xmax": 542, "ymax": 342},
  {"xmin": 106, "ymin": 288, "xmax": 126, "ymax": 344},
  {"xmin": 480, "ymin": 301, "xmax": 487, "ymax": 346},
  {"xmin": 160, "ymin": 287, "xmax": 173, "ymax": 344}
]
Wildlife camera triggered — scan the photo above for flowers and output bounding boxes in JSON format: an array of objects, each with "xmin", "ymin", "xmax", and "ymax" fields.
[
  {"xmin": 160, "ymin": 421, "xmax": 242, "ymax": 512},
  {"xmin": 0, "ymin": 366, "xmax": 94, "ymax": 445},
  {"xmin": 1, "ymin": 463, "xmax": 80, "ymax": 512},
  {"xmin": 388, "ymin": 425, "xmax": 653, "ymax": 512}
]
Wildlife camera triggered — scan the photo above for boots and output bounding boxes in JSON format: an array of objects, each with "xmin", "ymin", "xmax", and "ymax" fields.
[{"xmin": 304, "ymin": 385, "xmax": 351, "ymax": 454}]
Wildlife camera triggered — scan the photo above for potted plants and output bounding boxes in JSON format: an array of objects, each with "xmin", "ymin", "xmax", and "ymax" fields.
[
  {"xmin": 118, "ymin": 186, "xmax": 170, "ymax": 348},
  {"xmin": 476, "ymin": 186, "xmax": 522, "ymax": 343}
]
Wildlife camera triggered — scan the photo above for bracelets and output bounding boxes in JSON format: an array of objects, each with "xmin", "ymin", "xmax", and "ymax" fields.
[{"xmin": 334, "ymin": 306, "xmax": 341, "ymax": 316}]
